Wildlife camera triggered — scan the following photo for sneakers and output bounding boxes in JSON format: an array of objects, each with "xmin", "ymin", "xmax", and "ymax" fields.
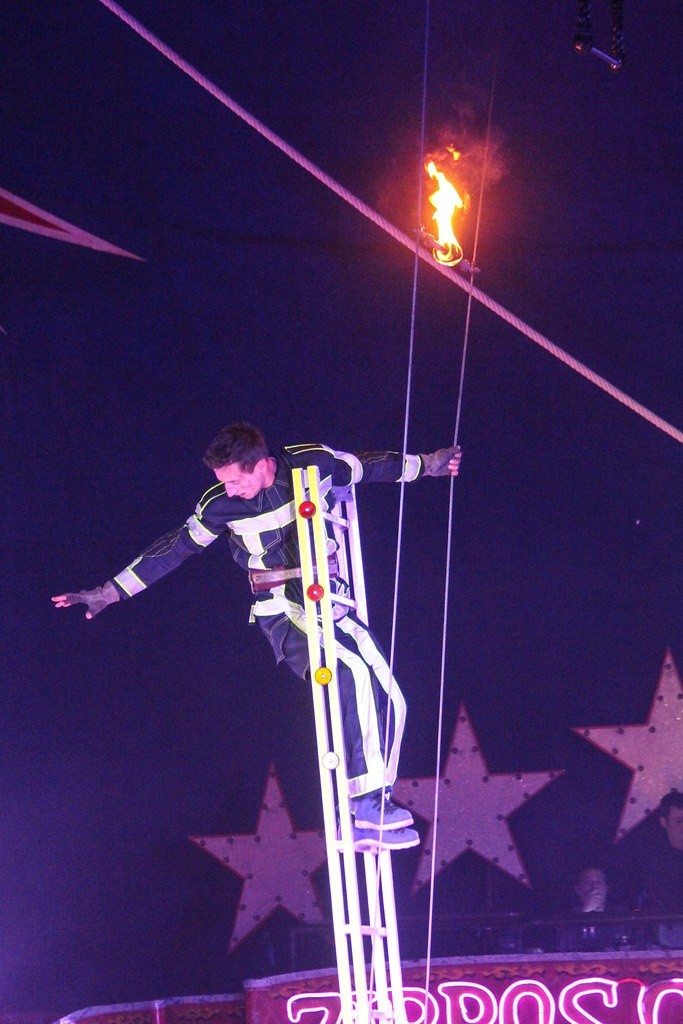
[
  {"xmin": 337, "ymin": 816, "xmax": 420, "ymax": 850},
  {"xmin": 355, "ymin": 794, "xmax": 414, "ymax": 831}
]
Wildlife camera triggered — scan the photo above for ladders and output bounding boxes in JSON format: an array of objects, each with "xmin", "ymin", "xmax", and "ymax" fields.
[{"xmin": 291, "ymin": 465, "xmax": 409, "ymax": 1024}]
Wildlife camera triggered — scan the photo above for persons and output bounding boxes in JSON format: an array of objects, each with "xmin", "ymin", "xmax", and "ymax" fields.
[{"xmin": 50, "ymin": 420, "xmax": 463, "ymax": 850}]
